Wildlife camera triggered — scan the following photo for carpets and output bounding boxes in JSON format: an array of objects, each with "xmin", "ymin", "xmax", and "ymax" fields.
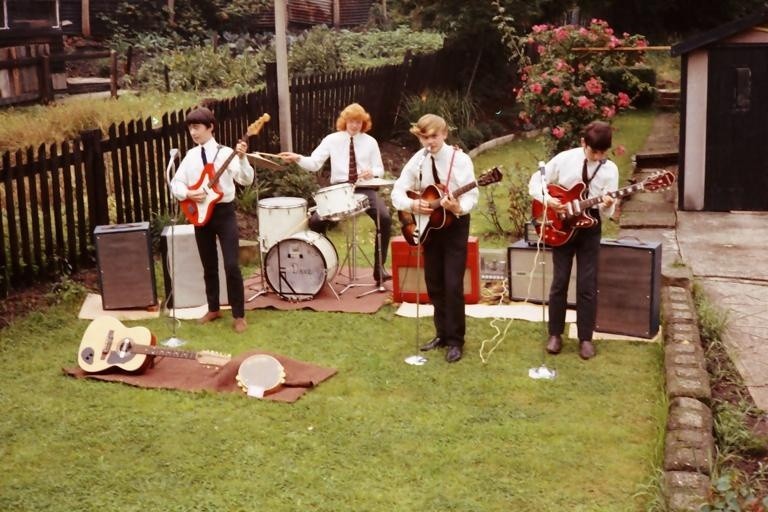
[
  {"xmin": 62, "ymin": 350, "xmax": 339, "ymax": 404},
  {"xmin": 395, "ymin": 300, "xmax": 662, "ymax": 343},
  {"xmin": 244, "ymin": 266, "xmax": 394, "ymax": 314}
]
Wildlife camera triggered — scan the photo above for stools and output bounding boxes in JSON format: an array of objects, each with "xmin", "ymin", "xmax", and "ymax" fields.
[{"xmin": 338, "ymin": 214, "xmax": 374, "ymax": 280}]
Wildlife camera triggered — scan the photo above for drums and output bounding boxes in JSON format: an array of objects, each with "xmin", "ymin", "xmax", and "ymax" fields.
[
  {"xmin": 314, "ymin": 183, "xmax": 369, "ymax": 221},
  {"xmin": 257, "ymin": 196, "xmax": 308, "ymax": 253},
  {"xmin": 263, "ymin": 231, "xmax": 338, "ymax": 301}
]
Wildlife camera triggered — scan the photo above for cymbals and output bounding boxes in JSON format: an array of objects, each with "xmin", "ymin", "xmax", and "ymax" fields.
[
  {"xmin": 357, "ymin": 179, "xmax": 395, "ymax": 187},
  {"xmin": 246, "ymin": 153, "xmax": 281, "ymax": 170}
]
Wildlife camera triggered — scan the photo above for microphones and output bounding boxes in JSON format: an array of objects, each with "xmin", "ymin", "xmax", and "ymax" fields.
[
  {"xmin": 538, "ymin": 160, "xmax": 545, "ymax": 176},
  {"xmin": 419, "ymin": 144, "xmax": 431, "ymax": 168}
]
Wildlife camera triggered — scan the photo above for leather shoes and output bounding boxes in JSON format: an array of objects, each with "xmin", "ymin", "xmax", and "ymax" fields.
[
  {"xmin": 232, "ymin": 316, "xmax": 248, "ymax": 334},
  {"xmin": 578, "ymin": 339, "xmax": 597, "ymax": 360},
  {"xmin": 197, "ymin": 308, "xmax": 224, "ymax": 324},
  {"xmin": 545, "ymin": 333, "xmax": 562, "ymax": 354},
  {"xmin": 444, "ymin": 344, "xmax": 463, "ymax": 363},
  {"xmin": 372, "ymin": 262, "xmax": 394, "ymax": 283},
  {"xmin": 418, "ymin": 335, "xmax": 445, "ymax": 352}
]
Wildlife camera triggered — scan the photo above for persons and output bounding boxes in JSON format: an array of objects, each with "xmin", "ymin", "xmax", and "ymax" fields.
[
  {"xmin": 169, "ymin": 107, "xmax": 255, "ymax": 334},
  {"xmin": 277, "ymin": 102, "xmax": 394, "ymax": 283},
  {"xmin": 390, "ymin": 113, "xmax": 480, "ymax": 364},
  {"xmin": 527, "ymin": 120, "xmax": 620, "ymax": 360}
]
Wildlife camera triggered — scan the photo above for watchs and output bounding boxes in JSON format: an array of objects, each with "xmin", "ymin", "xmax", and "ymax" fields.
[{"xmin": 454, "ymin": 212, "xmax": 462, "ymax": 216}]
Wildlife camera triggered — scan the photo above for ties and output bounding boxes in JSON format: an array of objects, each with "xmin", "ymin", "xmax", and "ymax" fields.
[
  {"xmin": 346, "ymin": 136, "xmax": 359, "ymax": 184},
  {"xmin": 429, "ymin": 155, "xmax": 442, "ymax": 187},
  {"xmin": 199, "ymin": 147, "xmax": 209, "ymax": 170},
  {"xmin": 581, "ymin": 159, "xmax": 590, "ymax": 200}
]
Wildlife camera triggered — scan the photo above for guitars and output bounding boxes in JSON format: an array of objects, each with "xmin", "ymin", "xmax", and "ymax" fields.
[
  {"xmin": 531, "ymin": 170, "xmax": 675, "ymax": 247},
  {"xmin": 179, "ymin": 113, "xmax": 270, "ymax": 225},
  {"xmin": 399, "ymin": 166, "xmax": 503, "ymax": 246},
  {"xmin": 79, "ymin": 314, "xmax": 231, "ymax": 375}
]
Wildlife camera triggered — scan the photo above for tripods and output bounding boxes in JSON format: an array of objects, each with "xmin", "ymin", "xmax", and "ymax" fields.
[
  {"xmin": 333, "ymin": 191, "xmax": 394, "ymax": 301},
  {"xmin": 245, "ymin": 163, "xmax": 276, "ymax": 303}
]
